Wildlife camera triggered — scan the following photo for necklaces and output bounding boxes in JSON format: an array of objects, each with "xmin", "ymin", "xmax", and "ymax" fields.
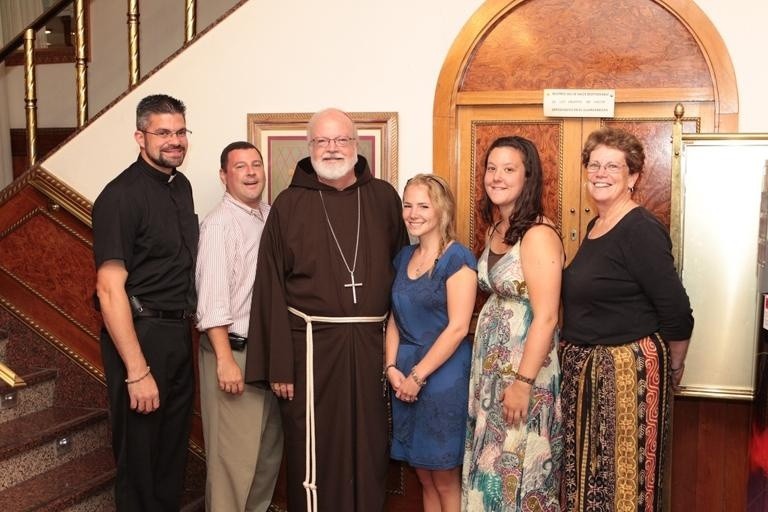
[
  {"xmin": 412, "ymin": 258, "xmax": 424, "ymax": 275},
  {"xmin": 319, "ymin": 186, "xmax": 364, "ymax": 303}
]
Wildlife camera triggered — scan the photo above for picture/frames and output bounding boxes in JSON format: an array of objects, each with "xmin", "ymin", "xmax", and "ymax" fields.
[{"xmin": 244, "ymin": 110, "xmax": 401, "ymax": 208}]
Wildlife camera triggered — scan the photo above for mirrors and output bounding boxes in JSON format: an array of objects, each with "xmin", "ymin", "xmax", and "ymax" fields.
[{"xmin": 5, "ymin": 0, "xmax": 92, "ymax": 68}]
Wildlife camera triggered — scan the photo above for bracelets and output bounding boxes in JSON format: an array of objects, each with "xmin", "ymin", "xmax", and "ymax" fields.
[
  {"xmin": 670, "ymin": 363, "xmax": 682, "ymax": 373},
  {"xmin": 384, "ymin": 364, "xmax": 397, "ymax": 371},
  {"xmin": 411, "ymin": 365, "xmax": 426, "ymax": 387},
  {"xmin": 515, "ymin": 372, "xmax": 534, "ymax": 385},
  {"xmin": 125, "ymin": 366, "xmax": 150, "ymax": 383}
]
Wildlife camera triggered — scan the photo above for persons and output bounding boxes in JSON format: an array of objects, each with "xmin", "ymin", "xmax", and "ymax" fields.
[
  {"xmin": 382, "ymin": 172, "xmax": 477, "ymax": 511},
  {"xmin": 459, "ymin": 136, "xmax": 562, "ymax": 511},
  {"xmin": 240, "ymin": 107, "xmax": 410, "ymax": 511},
  {"xmin": 193, "ymin": 142, "xmax": 286, "ymax": 511},
  {"xmin": 91, "ymin": 93, "xmax": 199, "ymax": 511},
  {"xmin": 557, "ymin": 129, "xmax": 695, "ymax": 511}
]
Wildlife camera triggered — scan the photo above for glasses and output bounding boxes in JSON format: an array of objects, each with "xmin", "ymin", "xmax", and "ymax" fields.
[
  {"xmin": 138, "ymin": 127, "xmax": 194, "ymax": 140},
  {"xmin": 309, "ymin": 136, "xmax": 359, "ymax": 147},
  {"xmin": 584, "ymin": 160, "xmax": 632, "ymax": 171}
]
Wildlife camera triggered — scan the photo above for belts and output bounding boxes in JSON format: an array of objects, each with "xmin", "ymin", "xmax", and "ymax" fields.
[{"xmin": 130, "ymin": 301, "xmax": 196, "ymax": 321}]
